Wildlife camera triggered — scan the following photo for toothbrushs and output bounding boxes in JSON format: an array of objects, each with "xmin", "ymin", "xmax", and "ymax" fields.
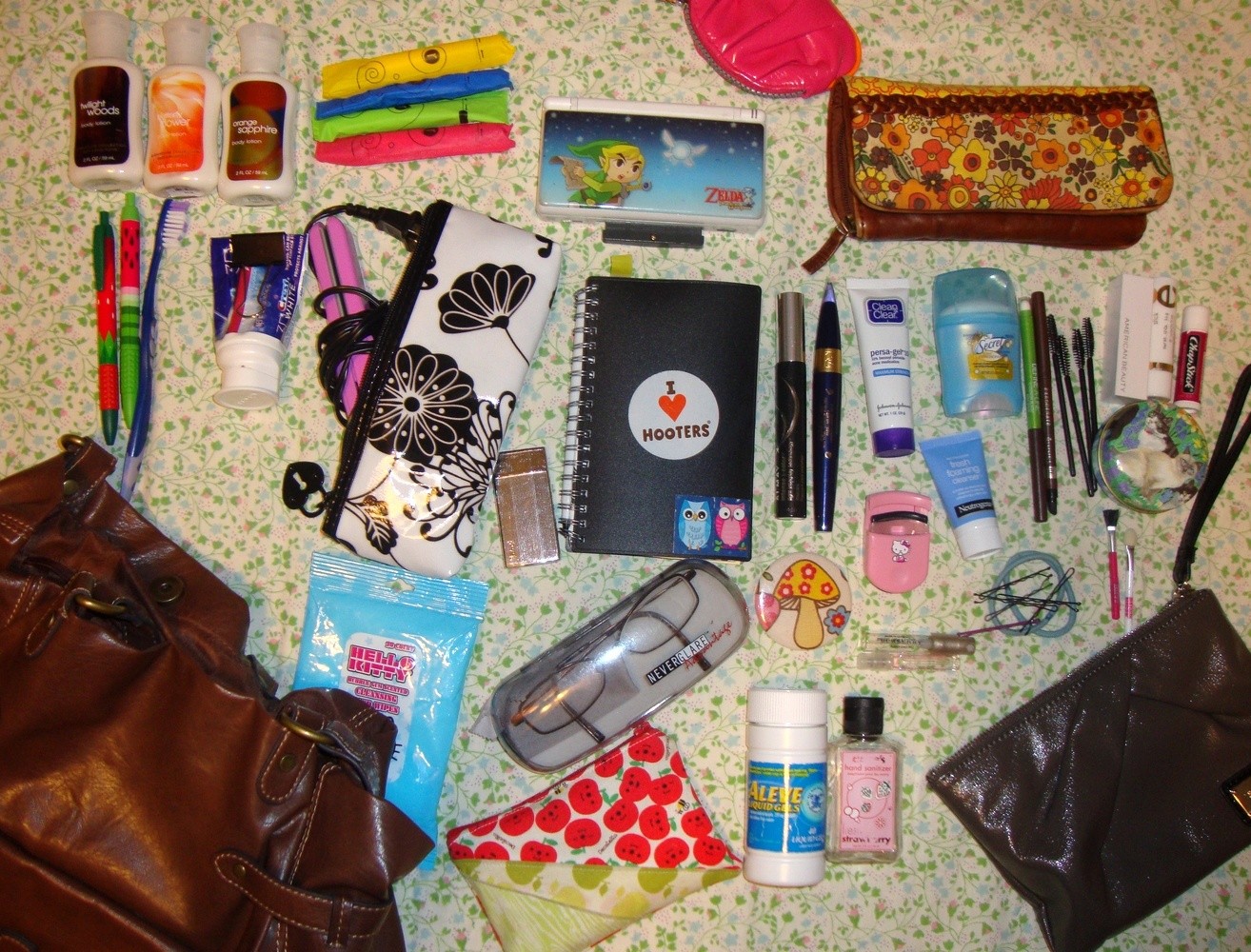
[{"xmin": 118, "ymin": 194, "xmax": 193, "ymax": 505}]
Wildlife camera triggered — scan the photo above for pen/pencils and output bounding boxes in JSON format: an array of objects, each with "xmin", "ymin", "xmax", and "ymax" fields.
[
  {"xmin": 1018, "ymin": 298, "xmax": 1047, "ymax": 522},
  {"xmin": 1031, "ymin": 290, "xmax": 1059, "ymax": 514},
  {"xmin": 814, "ymin": 281, "xmax": 841, "ymax": 531},
  {"xmin": 119, "ymin": 192, "xmax": 141, "ymax": 428},
  {"xmin": 95, "ymin": 210, "xmax": 117, "ymax": 445}
]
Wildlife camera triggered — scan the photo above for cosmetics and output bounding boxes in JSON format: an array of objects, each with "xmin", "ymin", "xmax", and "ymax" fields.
[
  {"xmin": 742, "ymin": 687, "xmax": 829, "ymax": 887},
  {"xmin": 1105, "ymin": 273, "xmax": 1208, "ymax": 415},
  {"xmin": 846, "ymin": 278, "xmax": 917, "ymax": 460},
  {"xmin": 862, "ymin": 491, "xmax": 932, "ymax": 595},
  {"xmin": 66, "ymin": 10, "xmax": 298, "ymax": 206},
  {"xmin": 918, "ymin": 429, "xmax": 1002, "ymax": 560},
  {"xmin": 933, "ymin": 267, "xmax": 1025, "ymax": 421}
]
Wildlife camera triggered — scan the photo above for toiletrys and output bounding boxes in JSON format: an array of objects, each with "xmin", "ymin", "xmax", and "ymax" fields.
[
  {"xmin": 843, "ymin": 278, "xmax": 917, "ymax": 458},
  {"xmin": 69, "ymin": 8, "xmax": 144, "ymax": 192},
  {"xmin": 208, "ymin": 231, "xmax": 313, "ymax": 409},
  {"xmin": 142, "ymin": 18, "xmax": 223, "ymax": 196},
  {"xmin": 932, "ymin": 266, "xmax": 1024, "ymax": 419},
  {"xmin": 218, "ymin": 21, "xmax": 299, "ymax": 208},
  {"xmin": 922, "ymin": 431, "xmax": 1002, "ymax": 561}
]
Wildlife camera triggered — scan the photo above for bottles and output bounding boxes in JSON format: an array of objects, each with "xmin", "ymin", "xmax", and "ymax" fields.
[{"xmin": 744, "ymin": 689, "xmax": 830, "ymax": 887}]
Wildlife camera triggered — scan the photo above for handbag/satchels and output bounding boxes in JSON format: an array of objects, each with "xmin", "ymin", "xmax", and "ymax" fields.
[
  {"xmin": 800, "ymin": 75, "xmax": 1173, "ymax": 274},
  {"xmin": 928, "ymin": 366, "xmax": 1251, "ymax": 952},
  {"xmin": 1, "ymin": 432, "xmax": 435, "ymax": 952}
]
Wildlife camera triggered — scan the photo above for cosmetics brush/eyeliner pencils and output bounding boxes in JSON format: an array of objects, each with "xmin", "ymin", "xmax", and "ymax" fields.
[
  {"xmin": 1021, "ymin": 289, "xmax": 1136, "ymax": 637},
  {"xmin": 774, "ymin": 280, "xmax": 844, "ymax": 533}
]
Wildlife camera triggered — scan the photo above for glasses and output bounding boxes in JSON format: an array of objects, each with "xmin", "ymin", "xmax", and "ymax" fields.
[{"xmin": 509, "ymin": 569, "xmax": 708, "ymax": 742}]
